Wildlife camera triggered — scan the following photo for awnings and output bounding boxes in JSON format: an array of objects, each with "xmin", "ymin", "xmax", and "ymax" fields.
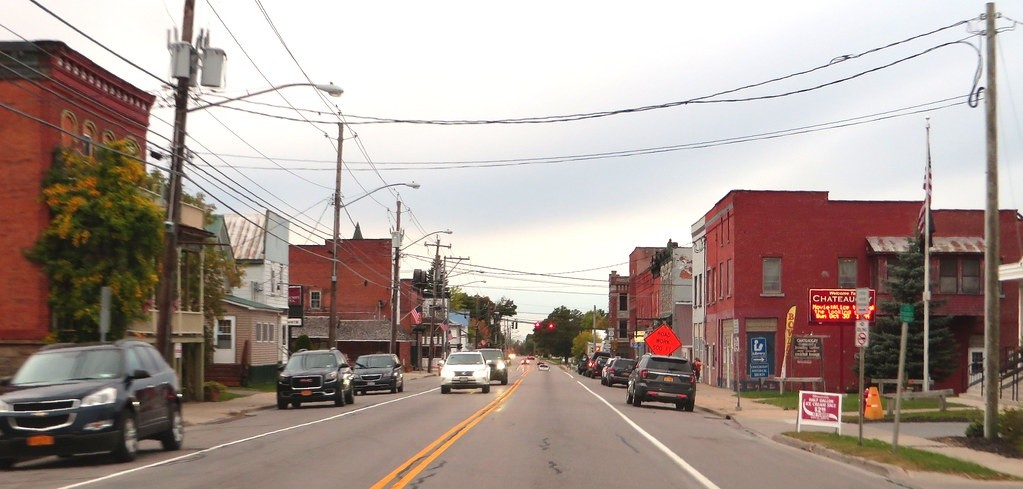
[{"xmin": 866, "ymin": 236, "xmax": 987, "ymax": 255}]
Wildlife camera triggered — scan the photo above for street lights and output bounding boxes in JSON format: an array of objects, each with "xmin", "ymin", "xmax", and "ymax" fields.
[
  {"xmin": 329, "ymin": 181, "xmax": 422, "ymax": 354},
  {"xmin": 156, "ymin": 80, "xmax": 343, "ymax": 365},
  {"xmin": 390, "ymin": 229, "xmax": 452, "ymax": 353},
  {"xmin": 428, "ymin": 270, "xmax": 486, "ymax": 371}
]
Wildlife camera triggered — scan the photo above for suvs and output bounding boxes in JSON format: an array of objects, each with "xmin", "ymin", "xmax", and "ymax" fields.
[
  {"xmin": 626, "ymin": 352, "xmax": 697, "ymax": 413},
  {"xmin": 277, "ymin": 346, "xmax": 356, "ymax": 409},
  {"xmin": 438, "ymin": 351, "xmax": 492, "ymax": 394},
  {"xmin": 477, "ymin": 349, "xmax": 510, "ymax": 385},
  {"xmin": 592, "ymin": 356, "xmax": 610, "ymax": 378},
  {"xmin": 0, "ymin": 337, "xmax": 183, "ymax": 468},
  {"xmin": 578, "ymin": 350, "xmax": 610, "ymax": 376},
  {"xmin": 601, "ymin": 357, "xmax": 636, "ymax": 387}
]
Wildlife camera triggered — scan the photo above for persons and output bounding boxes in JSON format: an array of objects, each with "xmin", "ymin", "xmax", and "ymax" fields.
[{"xmin": 694, "ymin": 358, "xmax": 703, "ymax": 383}]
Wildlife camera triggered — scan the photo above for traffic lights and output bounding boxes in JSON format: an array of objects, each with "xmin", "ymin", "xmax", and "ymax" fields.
[
  {"xmin": 596, "ymin": 348, "xmax": 599, "ymax": 350},
  {"xmin": 535, "ymin": 323, "xmax": 540, "ymax": 327},
  {"xmin": 511, "ymin": 321, "xmax": 513, "ymax": 329},
  {"xmin": 549, "ymin": 324, "xmax": 554, "ymax": 328},
  {"xmin": 591, "ymin": 347, "xmax": 594, "ymax": 352}
]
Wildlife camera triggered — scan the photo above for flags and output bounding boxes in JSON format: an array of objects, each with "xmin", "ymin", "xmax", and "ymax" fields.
[
  {"xmin": 441, "ymin": 320, "xmax": 448, "ymax": 331},
  {"xmin": 481, "ymin": 340, "xmax": 486, "ymax": 345},
  {"xmin": 917, "ymin": 144, "xmax": 933, "ymax": 240},
  {"xmin": 412, "ymin": 306, "xmax": 423, "ymax": 324},
  {"xmin": 448, "ymin": 331, "xmax": 452, "ymax": 340}
]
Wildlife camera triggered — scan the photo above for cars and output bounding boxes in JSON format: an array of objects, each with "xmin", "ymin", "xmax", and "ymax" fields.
[
  {"xmin": 355, "ymin": 353, "xmax": 403, "ymax": 394},
  {"xmin": 521, "ymin": 356, "xmax": 550, "ymax": 371}
]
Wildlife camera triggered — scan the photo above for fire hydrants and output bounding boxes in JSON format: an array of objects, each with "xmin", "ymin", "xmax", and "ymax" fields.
[{"xmin": 862, "ymin": 389, "xmax": 869, "ymax": 412}]
